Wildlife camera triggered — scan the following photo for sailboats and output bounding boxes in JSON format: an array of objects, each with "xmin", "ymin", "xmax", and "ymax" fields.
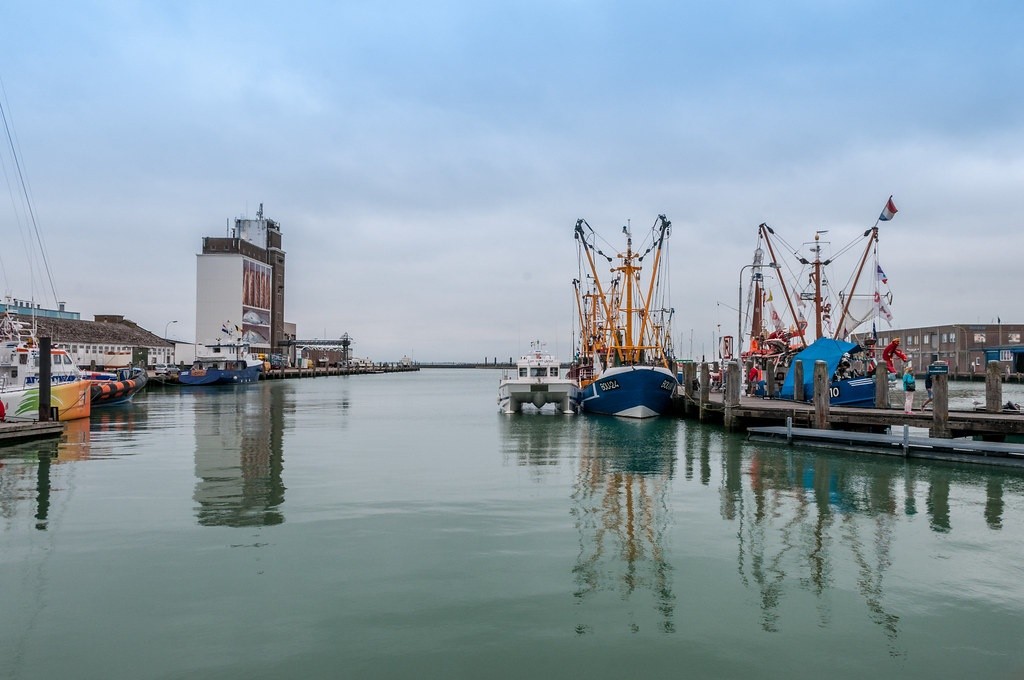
[{"xmin": 0, "ymin": 80, "xmax": 91, "ymax": 422}]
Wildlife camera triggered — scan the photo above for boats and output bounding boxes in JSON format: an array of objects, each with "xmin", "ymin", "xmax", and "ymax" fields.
[
  {"xmin": 498, "ymin": 340, "xmax": 580, "ymax": 416},
  {"xmin": 176, "ymin": 354, "xmax": 263, "ymax": 386},
  {"xmin": 572, "ymin": 214, "xmax": 678, "ymax": 417},
  {"xmin": 85, "ymin": 366, "xmax": 148, "ymax": 408},
  {"xmin": 743, "ymin": 193, "xmax": 908, "ymax": 411}
]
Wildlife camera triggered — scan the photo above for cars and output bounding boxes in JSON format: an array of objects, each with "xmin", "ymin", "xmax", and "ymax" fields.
[{"xmin": 154, "ymin": 363, "xmax": 179, "ymax": 377}]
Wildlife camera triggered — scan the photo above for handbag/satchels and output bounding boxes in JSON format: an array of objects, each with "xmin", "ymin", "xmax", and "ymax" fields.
[{"xmin": 906, "ymin": 381, "xmax": 915, "ymax": 392}]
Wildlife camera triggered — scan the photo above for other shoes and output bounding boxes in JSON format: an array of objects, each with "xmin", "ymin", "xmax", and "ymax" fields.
[
  {"xmin": 920, "ymin": 404, "xmax": 925, "ymax": 412},
  {"xmin": 904, "ymin": 411, "xmax": 914, "ymax": 414}
]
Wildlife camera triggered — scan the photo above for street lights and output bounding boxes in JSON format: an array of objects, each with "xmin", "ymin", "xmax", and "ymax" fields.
[
  {"xmin": 195, "ymin": 343, "xmax": 202, "ymax": 361},
  {"xmin": 163, "ymin": 321, "xmax": 178, "ymax": 363},
  {"xmin": 953, "ymin": 324, "xmax": 968, "ymax": 373}
]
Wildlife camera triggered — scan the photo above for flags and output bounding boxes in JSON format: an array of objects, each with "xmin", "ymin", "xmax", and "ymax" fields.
[
  {"xmin": 763, "ymin": 289, "xmax": 785, "ymax": 330},
  {"xmin": 797, "ymin": 309, "xmax": 808, "ymax": 331},
  {"xmin": 880, "ymin": 199, "xmax": 898, "ymax": 221},
  {"xmin": 874, "ymin": 261, "xmax": 888, "ymax": 284},
  {"xmin": 873, "ymin": 289, "xmax": 893, "ymax": 321},
  {"xmin": 792, "ymin": 287, "xmax": 806, "ymax": 308}
]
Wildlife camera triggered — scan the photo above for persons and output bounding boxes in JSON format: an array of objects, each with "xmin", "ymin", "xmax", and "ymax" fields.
[
  {"xmin": 822, "ymin": 303, "xmax": 834, "ymax": 336},
  {"xmin": 759, "ymin": 332, "xmax": 766, "ymax": 349},
  {"xmin": 751, "ymin": 336, "xmax": 759, "ymax": 350},
  {"xmin": 616, "ymin": 326, "xmax": 621, "ymax": 341},
  {"xmin": 920, "ymin": 374, "xmax": 934, "ymax": 411},
  {"xmin": 749, "ymin": 366, "xmax": 759, "ymax": 397},
  {"xmin": 127, "ymin": 362, "xmax": 133, "ymax": 370},
  {"xmin": 903, "ymin": 367, "xmax": 916, "ymax": 412}
]
